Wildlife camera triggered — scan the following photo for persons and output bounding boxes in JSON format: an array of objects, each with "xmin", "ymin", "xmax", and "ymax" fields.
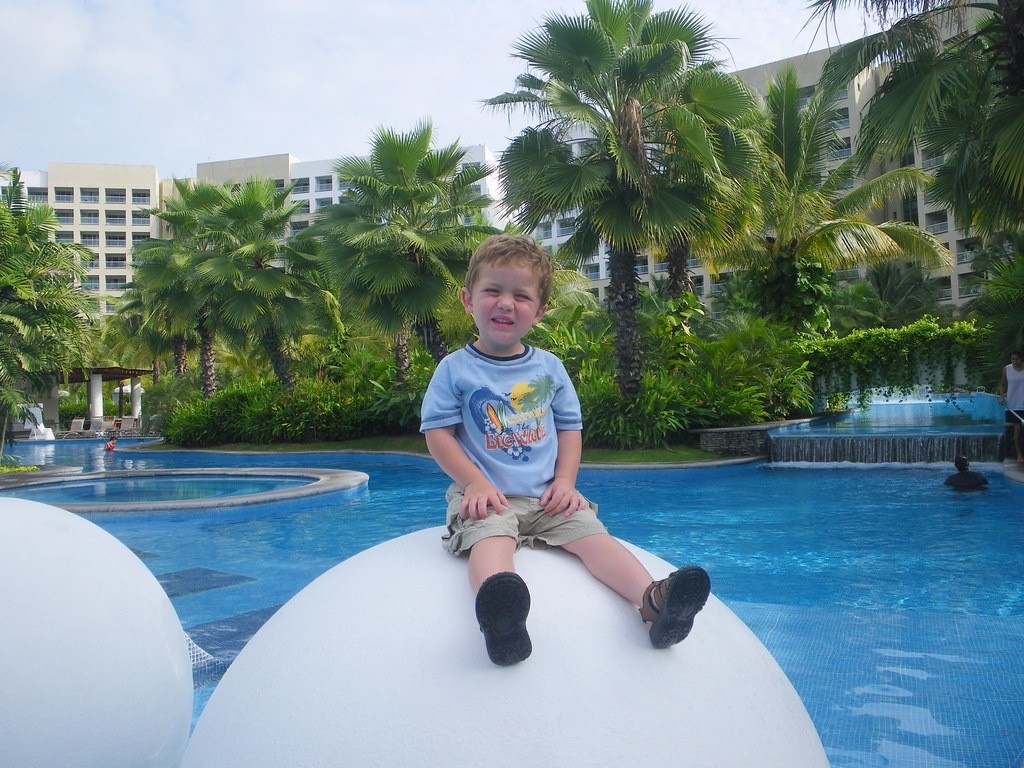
[
  {"xmin": 944, "ymin": 456, "xmax": 988, "ymax": 484},
  {"xmin": 999, "ymin": 350, "xmax": 1024, "ymax": 463},
  {"xmin": 104, "ymin": 436, "xmax": 118, "ymax": 451},
  {"xmin": 420, "ymin": 233, "xmax": 710, "ymax": 666}
]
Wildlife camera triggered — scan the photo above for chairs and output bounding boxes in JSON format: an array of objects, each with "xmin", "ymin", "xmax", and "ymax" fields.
[{"xmin": 52, "ymin": 416, "xmax": 142, "ymax": 440}]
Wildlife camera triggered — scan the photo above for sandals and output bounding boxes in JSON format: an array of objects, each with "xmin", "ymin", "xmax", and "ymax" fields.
[
  {"xmin": 640, "ymin": 565, "xmax": 711, "ymax": 649},
  {"xmin": 475, "ymin": 571, "xmax": 532, "ymax": 665}
]
